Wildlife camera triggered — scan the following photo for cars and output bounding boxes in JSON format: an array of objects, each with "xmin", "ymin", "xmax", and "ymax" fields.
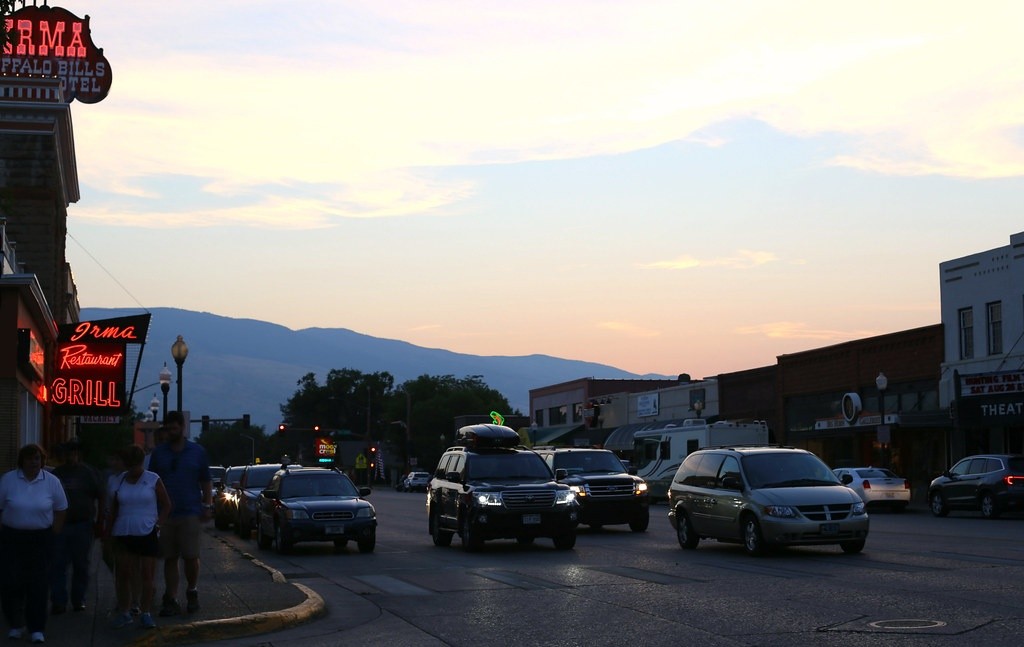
[
  {"xmin": 832, "ymin": 468, "xmax": 911, "ymax": 514},
  {"xmin": 257, "ymin": 464, "xmax": 377, "ymax": 556}
]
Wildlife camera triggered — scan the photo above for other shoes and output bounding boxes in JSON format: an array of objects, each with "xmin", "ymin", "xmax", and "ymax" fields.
[
  {"xmin": 72, "ymin": 602, "xmax": 86, "ymax": 612},
  {"xmin": 129, "ymin": 603, "xmax": 142, "ymax": 615},
  {"xmin": 50, "ymin": 603, "xmax": 67, "ymax": 616},
  {"xmin": 158, "ymin": 598, "xmax": 182, "ymax": 618},
  {"xmin": 186, "ymin": 589, "xmax": 200, "ymax": 615}
]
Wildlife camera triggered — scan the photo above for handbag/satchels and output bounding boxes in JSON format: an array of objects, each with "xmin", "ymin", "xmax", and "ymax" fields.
[{"xmin": 101, "ymin": 474, "xmax": 125, "ymax": 541}]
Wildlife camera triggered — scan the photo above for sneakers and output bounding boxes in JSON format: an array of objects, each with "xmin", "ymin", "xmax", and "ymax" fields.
[
  {"xmin": 138, "ymin": 614, "xmax": 157, "ymax": 629},
  {"xmin": 31, "ymin": 632, "xmax": 45, "ymax": 643},
  {"xmin": 7, "ymin": 629, "xmax": 22, "ymax": 639},
  {"xmin": 109, "ymin": 613, "xmax": 134, "ymax": 629}
]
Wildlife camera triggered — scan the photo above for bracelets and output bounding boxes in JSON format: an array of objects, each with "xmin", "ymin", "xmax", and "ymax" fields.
[
  {"xmin": 204, "ymin": 503, "xmax": 212, "ymax": 509},
  {"xmin": 155, "ymin": 524, "xmax": 161, "ymax": 529}
]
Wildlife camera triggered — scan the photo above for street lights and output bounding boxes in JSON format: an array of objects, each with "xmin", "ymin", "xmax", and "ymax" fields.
[
  {"xmin": 876, "ymin": 372, "xmax": 887, "ymax": 459},
  {"xmin": 150, "ymin": 393, "xmax": 160, "ymax": 422},
  {"xmin": 239, "ymin": 434, "xmax": 255, "ymax": 465},
  {"xmin": 170, "ymin": 334, "xmax": 188, "ymax": 411},
  {"xmin": 158, "ymin": 361, "xmax": 173, "ymax": 423}
]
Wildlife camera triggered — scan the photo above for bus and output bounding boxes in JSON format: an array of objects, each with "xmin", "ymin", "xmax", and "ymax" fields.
[{"xmin": 632, "ymin": 419, "xmax": 769, "ymax": 505}]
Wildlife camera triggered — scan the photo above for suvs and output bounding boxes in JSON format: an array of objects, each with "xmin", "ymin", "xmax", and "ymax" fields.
[
  {"xmin": 426, "ymin": 424, "xmax": 581, "ymax": 555},
  {"xmin": 403, "ymin": 471, "xmax": 430, "ymax": 493},
  {"xmin": 207, "ymin": 463, "xmax": 227, "ymax": 491},
  {"xmin": 231, "ymin": 463, "xmax": 303, "ymax": 541},
  {"xmin": 928, "ymin": 454, "xmax": 1024, "ymax": 521},
  {"xmin": 668, "ymin": 443, "xmax": 870, "ymax": 558},
  {"xmin": 532, "ymin": 446, "xmax": 649, "ymax": 533},
  {"xmin": 212, "ymin": 465, "xmax": 247, "ymax": 531}
]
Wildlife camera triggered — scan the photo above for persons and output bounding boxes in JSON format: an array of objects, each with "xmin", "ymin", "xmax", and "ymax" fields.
[
  {"xmin": 0, "ymin": 442, "xmax": 69, "ymax": 642},
  {"xmin": 47, "ymin": 408, "xmax": 215, "ymax": 630},
  {"xmin": 868, "ymin": 460, "xmax": 896, "ymax": 475}
]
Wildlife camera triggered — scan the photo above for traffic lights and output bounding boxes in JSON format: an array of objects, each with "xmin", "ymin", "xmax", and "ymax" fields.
[
  {"xmin": 314, "ymin": 424, "xmax": 319, "ymax": 434},
  {"xmin": 279, "ymin": 424, "xmax": 285, "ymax": 437}
]
